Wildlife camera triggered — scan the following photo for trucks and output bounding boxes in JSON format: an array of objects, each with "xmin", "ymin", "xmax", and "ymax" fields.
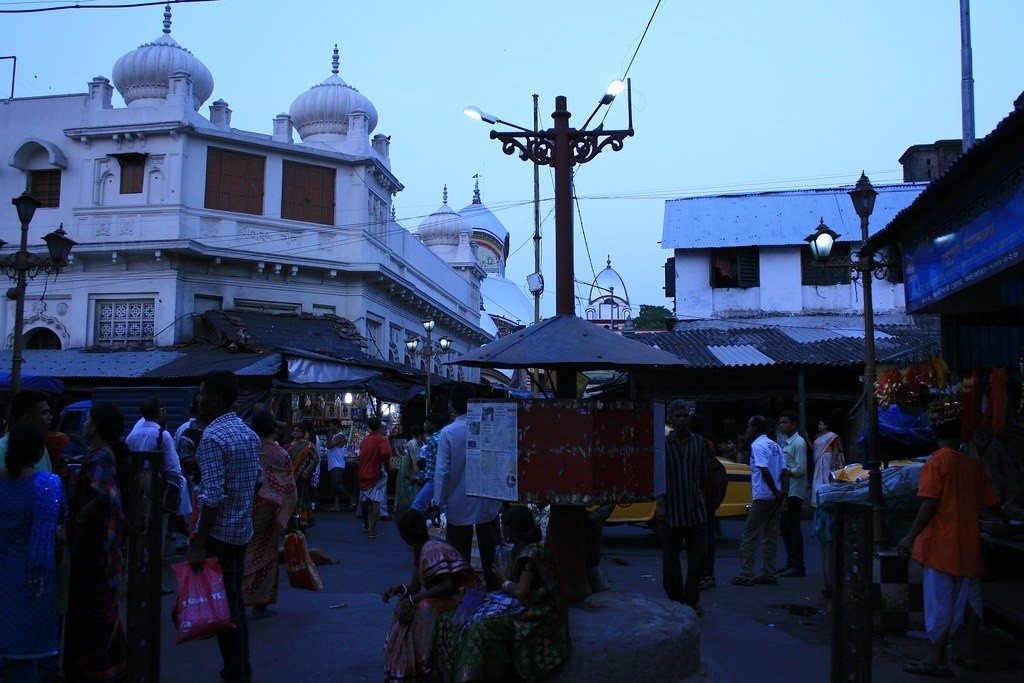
[{"xmin": 54, "ymin": 386, "xmax": 201, "ymax": 478}]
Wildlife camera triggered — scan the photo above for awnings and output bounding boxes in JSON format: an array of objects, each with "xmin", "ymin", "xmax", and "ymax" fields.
[
  {"xmin": 858, "ymin": 91, "xmax": 1024, "ymax": 316},
  {"xmin": 0, "ymin": 347, "xmax": 280, "ymax": 380},
  {"xmin": 210, "ymin": 311, "xmax": 506, "ymax": 398},
  {"xmin": 782, "ymin": 314, "xmax": 786, "ymax": 320},
  {"xmin": 624, "ymin": 323, "xmax": 941, "ymax": 369}
]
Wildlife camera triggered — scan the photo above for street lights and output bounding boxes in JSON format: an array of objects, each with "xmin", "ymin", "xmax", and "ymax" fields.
[
  {"xmin": 462, "ymin": 79, "xmax": 627, "ymax": 401},
  {"xmin": 803, "ymin": 170, "xmax": 890, "ymax": 554},
  {"xmin": 0, "ymin": 185, "xmax": 79, "ymax": 396},
  {"xmin": 403, "ymin": 314, "xmax": 455, "ymax": 415}
]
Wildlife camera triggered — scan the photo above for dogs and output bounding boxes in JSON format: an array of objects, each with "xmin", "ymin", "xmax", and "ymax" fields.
[{"xmin": 278, "ymin": 547, "xmax": 341, "ymax": 564}]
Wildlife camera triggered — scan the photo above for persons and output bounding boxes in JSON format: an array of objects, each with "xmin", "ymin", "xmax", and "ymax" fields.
[
  {"xmin": 731, "ymin": 411, "xmax": 845, "ymax": 587},
  {"xmin": 893, "ymin": 406, "xmax": 1011, "ymax": 676},
  {"xmin": 693, "ymin": 409, "xmax": 752, "ymax": 481},
  {"xmin": 656, "ymin": 400, "xmax": 729, "ymax": 616},
  {"xmin": 0, "ymin": 370, "xmax": 614, "ymax": 683}
]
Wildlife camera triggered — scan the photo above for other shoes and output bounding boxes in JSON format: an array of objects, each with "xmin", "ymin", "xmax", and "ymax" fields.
[
  {"xmin": 363, "ymin": 521, "xmax": 371, "ymax": 532},
  {"xmin": 251, "ymin": 606, "xmax": 279, "ymax": 619},
  {"xmin": 731, "ymin": 576, "xmax": 755, "ymax": 587},
  {"xmin": 777, "ymin": 568, "xmax": 807, "ymax": 577},
  {"xmin": 698, "ymin": 575, "xmax": 715, "ymax": 590},
  {"xmin": 688, "ymin": 601, "xmax": 704, "ymax": 616},
  {"xmin": 753, "ymin": 576, "xmax": 778, "ymax": 585},
  {"xmin": 369, "ymin": 533, "xmax": 379, "ymax": 537}
]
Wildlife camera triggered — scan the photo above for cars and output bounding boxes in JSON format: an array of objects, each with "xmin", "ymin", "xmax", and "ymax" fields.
[{"xmin": 587, "ymin": 424, "xmax": 754, "ymax": 547}]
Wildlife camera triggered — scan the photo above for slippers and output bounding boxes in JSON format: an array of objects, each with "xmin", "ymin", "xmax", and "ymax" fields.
[
  {"xmin": 903, "ymin": 658, "xmax": 954, "ymax": 678},
  {"xmin": 951, "ymin": 654, "xmax": 982, "ymax": 672}
]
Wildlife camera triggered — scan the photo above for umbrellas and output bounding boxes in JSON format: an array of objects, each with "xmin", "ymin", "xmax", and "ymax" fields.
[{"xmin": 441, "ymin": 313, "xmax": 691, "ymax": 398}]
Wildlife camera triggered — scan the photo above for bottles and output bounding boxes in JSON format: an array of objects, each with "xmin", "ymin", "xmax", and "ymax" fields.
[{"xmin": 494, "ymin": 545, "xmax": 506, "ymax": 567}]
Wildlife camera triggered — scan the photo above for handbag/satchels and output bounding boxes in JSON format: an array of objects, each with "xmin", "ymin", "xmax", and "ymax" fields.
[
  {"xmin": 170, "ymin": 556, "xmax": 237, "ymax": 643},
  {"xmin": 284, "ymin": 530, "xmax": 324, "ymax": 591}
]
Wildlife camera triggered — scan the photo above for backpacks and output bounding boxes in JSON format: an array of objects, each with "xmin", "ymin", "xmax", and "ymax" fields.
[{"xmin": 705, "ymin": 458, "xmax": 728, "ymax": 512}]
